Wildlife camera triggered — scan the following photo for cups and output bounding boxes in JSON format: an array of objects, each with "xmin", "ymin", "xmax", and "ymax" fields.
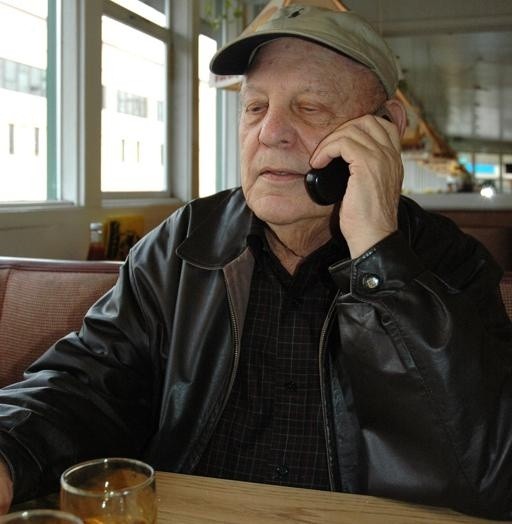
[
  {"xmin": 55, "ymin": 454, "xmax": 157, "ymax": 523},
  {"xmin": 1, "ymin": 508, "xmax": 89, "ymax": 524}
]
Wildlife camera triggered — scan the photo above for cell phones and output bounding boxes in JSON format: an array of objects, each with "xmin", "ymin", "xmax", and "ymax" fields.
[{"xmin": 305, "ymin": 107, "xmax": 394, "ymax": 205}]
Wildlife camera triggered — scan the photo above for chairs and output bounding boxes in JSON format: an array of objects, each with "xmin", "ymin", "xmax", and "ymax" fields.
[
  {"xmin": 1, "ymin": 256, "xmax": 136, "ymax": 389},
  {"xmin": 433, "ymin": 209, "xmax": 511, "ymax": 318}
]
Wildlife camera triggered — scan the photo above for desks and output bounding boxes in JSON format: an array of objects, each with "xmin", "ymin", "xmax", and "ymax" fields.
[{"xmin": 1, "ymin": 451, "xmax": 512, "ymax": 521}]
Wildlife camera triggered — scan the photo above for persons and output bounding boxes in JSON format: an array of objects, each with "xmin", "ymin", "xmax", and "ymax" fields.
[
  {"xmin": 455, "ymin": 166, "xmax": 473, "ymax": 192},
  {"xmin": 0, "ymin": 0, "xmax": 512, "ymax": 517}
]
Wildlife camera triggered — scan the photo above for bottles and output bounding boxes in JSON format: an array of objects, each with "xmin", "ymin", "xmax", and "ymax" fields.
[{"xmin": 86, "ymin": 221, "xmax": 107, "ymax": 260}]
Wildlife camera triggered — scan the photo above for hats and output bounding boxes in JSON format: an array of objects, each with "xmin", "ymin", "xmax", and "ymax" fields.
[{"xmin": 210, "ymin": 0, "xmax": 397, "ymax": 99}]
